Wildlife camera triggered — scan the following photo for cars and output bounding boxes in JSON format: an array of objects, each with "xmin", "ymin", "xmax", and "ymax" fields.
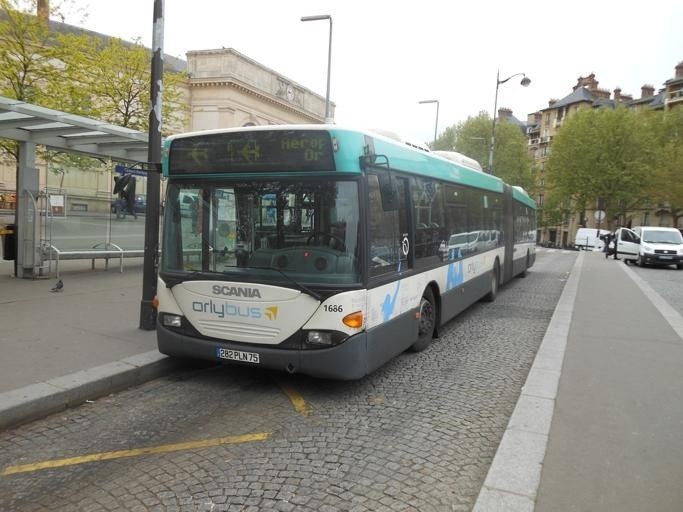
[
  {"xmin": 111, "ymin": 195, "xmax": 146, "ymax": 215},
  {"xmin": 177, "ymin": 192, "xmax": 199, "ymax": 218}
]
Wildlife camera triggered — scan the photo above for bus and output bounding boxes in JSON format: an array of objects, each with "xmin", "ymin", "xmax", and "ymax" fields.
[{"xmin": 153, "ymin": 123, "xmax": 540, "ymax": 390}]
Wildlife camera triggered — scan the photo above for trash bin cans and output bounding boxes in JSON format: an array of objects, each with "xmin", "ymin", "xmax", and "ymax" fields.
[{"xmin": 0, "ymin": 224, "xmax": 15, "ymax": 260}]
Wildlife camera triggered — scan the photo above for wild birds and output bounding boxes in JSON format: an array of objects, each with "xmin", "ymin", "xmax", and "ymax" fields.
[{"xmin": 51, "ymin": 279, "xmax": 63, "ymax": 290}]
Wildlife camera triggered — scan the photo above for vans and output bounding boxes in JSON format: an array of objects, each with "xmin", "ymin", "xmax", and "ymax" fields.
[
  {"xmin": 435, "ymin": 228, "xmax": 501, "ymax": 262},
  {"xmin": 573, "ymin": 227, "xmax": 614, "ymax": 254},
  {"xmin": 616, "ymin": 226, "xmax": 682, "ymax": 270}
]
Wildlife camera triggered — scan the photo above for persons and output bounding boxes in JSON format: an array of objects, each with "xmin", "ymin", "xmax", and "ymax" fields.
[
  {"xmin": 604, "ymin": 230, "xmax": 620, "ymax": 259},
  {"xmin": 335, "ymin": 210, "xmax": 389, "ymax": 267}
]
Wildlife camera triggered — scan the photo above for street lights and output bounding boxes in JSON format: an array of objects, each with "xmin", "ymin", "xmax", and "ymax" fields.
[
  {"xmin": 299, "ymin": 13, "xmax": 332, "ymax": 120},
  {"xmin": 487, "ymin": 71, "xmax": 531, "ymax": 175},
  {"xmin": 417, "ymin": 99, "xmax": 440, "ymax": 149}
]
